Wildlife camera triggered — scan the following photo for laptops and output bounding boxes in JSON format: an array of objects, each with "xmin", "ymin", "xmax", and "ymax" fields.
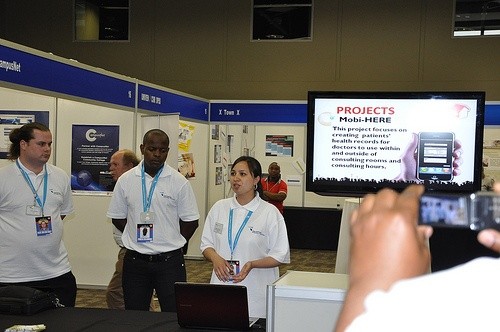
[{"xmin": 175, "ymin": 282, "xmax": 260, "ymax": 330}]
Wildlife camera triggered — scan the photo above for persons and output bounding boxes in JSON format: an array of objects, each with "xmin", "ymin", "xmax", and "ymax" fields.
[
  {"xmin": 333, "ymin": 182, "xmax": 500, "ymax": 332},
  {"xmin": 106, "ymin": 129, "xmax": 200, "ymax": 312},
  {"xmin": 106, "ymin": 149, "xmax": 156, "ymax": 311},
  {"xmin": 260, "ymin": 162, "xmax": 287, "ymax": 215},
  {"xmin": 0, "ymin": 122, "xmax": 77, "ymax": 308},
  {"xmin": 200, "ymin": 156, "xmax": 290, "ymax": 318}
]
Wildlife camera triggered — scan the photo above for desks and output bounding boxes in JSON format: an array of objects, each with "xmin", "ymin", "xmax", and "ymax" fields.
[
  {"xmin": 284, "ymin": 206, "xmax": 342, "ymax": 250},
  {"xmin": 0, "ymin": 306, "xmax": 266, "ymax": 332}
]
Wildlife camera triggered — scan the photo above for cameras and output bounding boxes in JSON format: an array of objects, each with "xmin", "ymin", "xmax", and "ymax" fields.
[{"xmin": 419, "ymin": 191, "xmax": 500, "ymax": 230}]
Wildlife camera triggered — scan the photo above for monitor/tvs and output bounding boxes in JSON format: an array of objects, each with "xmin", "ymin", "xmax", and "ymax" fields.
[{"xmin": 306, "ymin": 91, "xmax": 486, "ymax": 192}]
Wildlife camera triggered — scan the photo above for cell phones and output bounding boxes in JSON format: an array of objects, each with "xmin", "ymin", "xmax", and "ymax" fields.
[{"xmin": 418, "ymin": 131, "xmax": 454, "ymax": 182}]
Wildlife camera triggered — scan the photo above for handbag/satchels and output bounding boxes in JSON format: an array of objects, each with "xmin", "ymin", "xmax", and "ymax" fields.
[{"xmin": 0, "ymin": 286, "xmax": 55, "ymax": 315}]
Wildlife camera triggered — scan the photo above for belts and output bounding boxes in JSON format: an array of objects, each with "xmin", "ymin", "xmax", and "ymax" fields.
[{"xmin": 126, "ymin": 247, "xmax": 185, "ymax": 262}]
